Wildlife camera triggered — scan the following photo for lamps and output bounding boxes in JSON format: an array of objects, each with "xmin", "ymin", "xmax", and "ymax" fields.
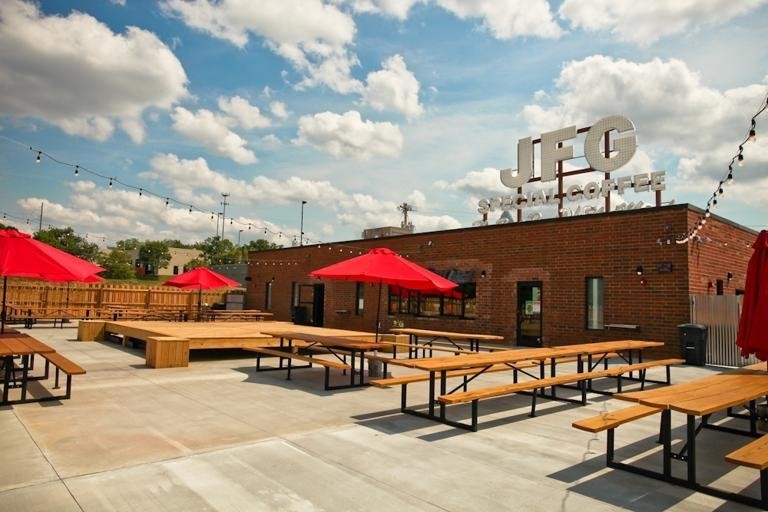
[
  {"xmin": 727, "ymin": 272, "xmax": 732, "ymax": 281},
  {"xmin": 636, "ymin": 266, "xmax": 643, "ymax": 276},
  {"xmin": 481, "ymin": 270, "xmax": 486, "ymax": 278}
]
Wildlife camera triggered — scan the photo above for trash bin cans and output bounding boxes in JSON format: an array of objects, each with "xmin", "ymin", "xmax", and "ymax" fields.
[{"xmin": 677, "ymin": 324, "xmax": 706, "ymax": 366}]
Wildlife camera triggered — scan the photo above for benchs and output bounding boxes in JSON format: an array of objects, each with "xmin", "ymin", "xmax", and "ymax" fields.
[
  {"xmin": 79, "ymin": 303, "xmax": 277, "ymax": 322},
  {"xmin": 371, "ymin": 340, "xmax": 685, "ymax": 432},
  {"xmin": 0, "ymin": 298, "xmax": 78, "ymax": 406},
  {"xmin": 240, "ymin": 324, "xmax": 506, "ymax": 391},
  {"xmin": 571, "ymin": 356, "xmax": 768, "ymax": 511}
]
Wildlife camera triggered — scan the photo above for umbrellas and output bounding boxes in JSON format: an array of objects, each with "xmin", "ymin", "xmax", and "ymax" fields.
[
  {"xmin": 735, "ymin": 228, "xmax": 768, "ymax": 362},
  {"xmin": 306, "ymin": 247, "xmax": 463, "ymax": 343},
  {"xmin": 161, "ymin": 266, "xmax": 240, "ymax": 311},
  {"xmin": 0, "ymin": 228, "xmax": 106, "ymax": 333}
]
